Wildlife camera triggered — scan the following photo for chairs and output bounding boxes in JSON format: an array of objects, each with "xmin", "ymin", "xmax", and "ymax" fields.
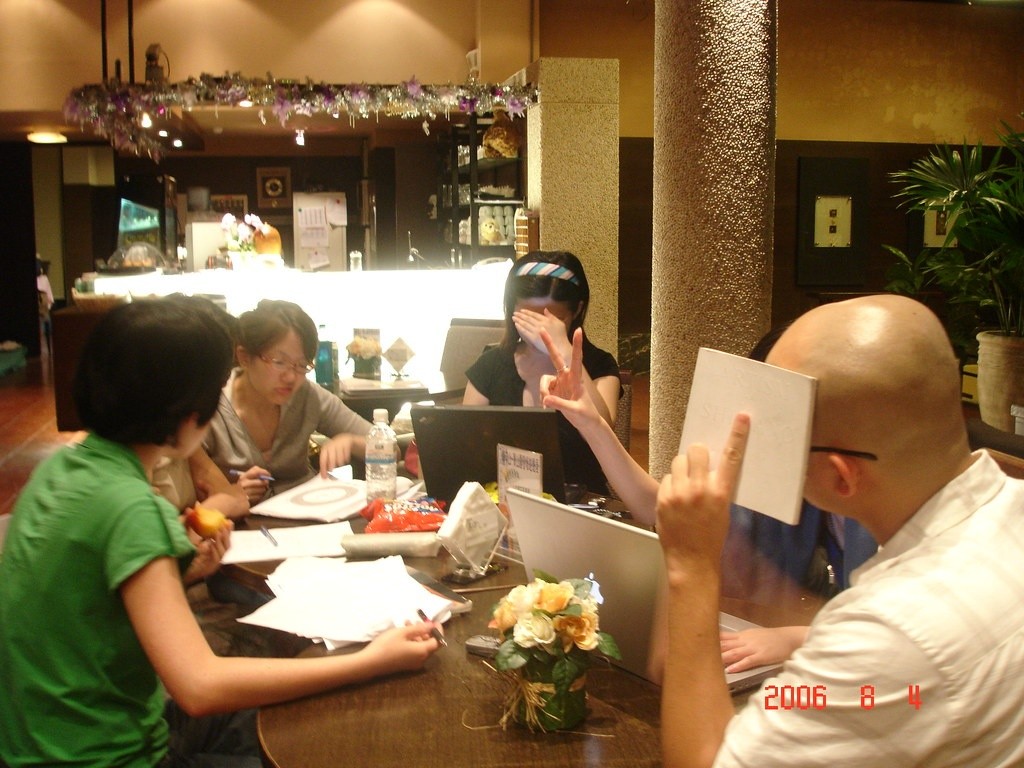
[{"xmin": 433, "ymin": 317, "xmax": 512, "ymax": 406}]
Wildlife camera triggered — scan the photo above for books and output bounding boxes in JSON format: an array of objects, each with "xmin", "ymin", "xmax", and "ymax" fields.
[
  {"xmin": 405, "ymin": 562, "xmax": 473, "ymax": 620},
  {"xmin": 677, "ymin": 346, "xmax": 816, "ymax": 525}
]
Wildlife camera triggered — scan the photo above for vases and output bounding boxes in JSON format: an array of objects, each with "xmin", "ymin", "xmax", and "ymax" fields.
[
  {"xmin": 351, "ymin": 356, "xmax": 378, "ymax": 374},
  {"xmin": 516, "ymin": 652, "xmax": 590, "ymax": 732}
]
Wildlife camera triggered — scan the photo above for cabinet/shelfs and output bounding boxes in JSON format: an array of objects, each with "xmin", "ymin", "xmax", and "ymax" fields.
[{"xmin": 436, "ymin": 110, "xmax": 525, "ymax": 269}]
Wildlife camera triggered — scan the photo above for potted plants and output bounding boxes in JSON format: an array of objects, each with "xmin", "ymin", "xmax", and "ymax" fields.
[{"xmin": 878, "ymin": 110, "xmax": 1024, "ymax": 434}]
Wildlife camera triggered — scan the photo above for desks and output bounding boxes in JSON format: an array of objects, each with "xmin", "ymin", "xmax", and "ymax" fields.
[
  {"xmin": 307, "ymin": 372, "xmax": 430, "ymax": 428},
  {"xmin": 219, "ymin": 464, "xmax": 828, "ymax": 768}
]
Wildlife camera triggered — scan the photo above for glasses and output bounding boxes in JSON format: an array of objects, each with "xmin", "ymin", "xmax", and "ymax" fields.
[{"xmin": 258, "ymin": 355, "xmax": 315, "ymax": 374}]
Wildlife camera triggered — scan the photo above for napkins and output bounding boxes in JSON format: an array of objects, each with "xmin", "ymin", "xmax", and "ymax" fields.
[
  {"xmin": 383, "ymin": 337, "xmax": 416, "ymax": 373},
  {"xmin": 436, "ymin": 482, "xmax": 507, "ymax": 569}
]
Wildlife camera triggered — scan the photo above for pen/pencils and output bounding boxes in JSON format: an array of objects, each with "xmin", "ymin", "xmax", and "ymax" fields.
[
  {"xmin": 261, "ymin": 524, "xmax": 279, "ymax": 548},
  {"xmin": 230, "ymin": 469, "xmax": 275, "ymax": 481},
  {"xmin": 416, "ymin": 608, "xmax": 449, "ymax": 649},
  {"xmin": 325, "ymin": 472, "xmax": 338, "ymax": 480}
]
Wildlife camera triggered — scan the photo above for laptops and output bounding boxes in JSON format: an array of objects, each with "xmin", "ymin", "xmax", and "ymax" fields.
[
  {"xmin": 411, "ymin": 403, "xmax": 588, "ymax": 505},
  {"xmin": 507, "ymin": 483, "xmax": 791, "ymax": 696}
]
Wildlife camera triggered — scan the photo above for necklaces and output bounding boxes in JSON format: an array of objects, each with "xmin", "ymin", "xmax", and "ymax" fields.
[{"xmin": 252, "ymin": 403, "xmax": 281, "ymax": 441}]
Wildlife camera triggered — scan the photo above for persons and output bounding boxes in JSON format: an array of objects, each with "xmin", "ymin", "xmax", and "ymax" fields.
[
  {"xmin": 0, "ymin": 299, "xmax": 445, "ymax": 768},
  {"xmin": 151, "ymin": 291, "xmax": 273, "ymax": 657},
  {"xmin": 461, "ymin": 249, "xmax": 625, "ymax": 492},
  {"xmin": 34, "ymin": 258, "xmax": 54, "ymax": 352},
  {"xmin": 654, "ymin": 294, "xmax": 1024, "ymax": 768},
  {"xmin": 203, "ymin": 298, "xmax": 403, "ymax": 510}
]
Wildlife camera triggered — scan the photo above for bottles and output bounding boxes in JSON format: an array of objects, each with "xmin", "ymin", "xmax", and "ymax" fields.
[{"xmin": 366, "ymin": 408, "xmax": 397, "ymax": 503}]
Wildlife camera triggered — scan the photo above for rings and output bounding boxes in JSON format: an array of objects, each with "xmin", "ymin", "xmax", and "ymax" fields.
[
  {"xmin": 247, "ymin": 495, "xmax": 250, "ymax": 500},
  {"xmin": 556, "ymin": 365, "xmax": 568, "ymax": 373},
  {"xmin": 209, "ymin": 537, "xmax": 216, "ymax": 543}
]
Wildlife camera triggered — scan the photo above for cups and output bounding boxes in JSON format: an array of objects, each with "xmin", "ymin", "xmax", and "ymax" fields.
[{"xmin": 459, "ymin": 205, "xmax": 513, "ymax": 244}]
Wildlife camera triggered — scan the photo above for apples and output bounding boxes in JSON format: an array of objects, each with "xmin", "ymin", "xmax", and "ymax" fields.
[{"xmin": 190, "ymin": 505, "xmax": 226, "ymax": 537}]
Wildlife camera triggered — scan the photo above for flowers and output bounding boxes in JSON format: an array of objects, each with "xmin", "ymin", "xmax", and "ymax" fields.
[
  {"xmin": 346, "ymin": 337, "xmax": 383, "ymax": 370},
  {"xmin": 488, "ymin": 566, "xmax": 624, "ymax": 695}
]
